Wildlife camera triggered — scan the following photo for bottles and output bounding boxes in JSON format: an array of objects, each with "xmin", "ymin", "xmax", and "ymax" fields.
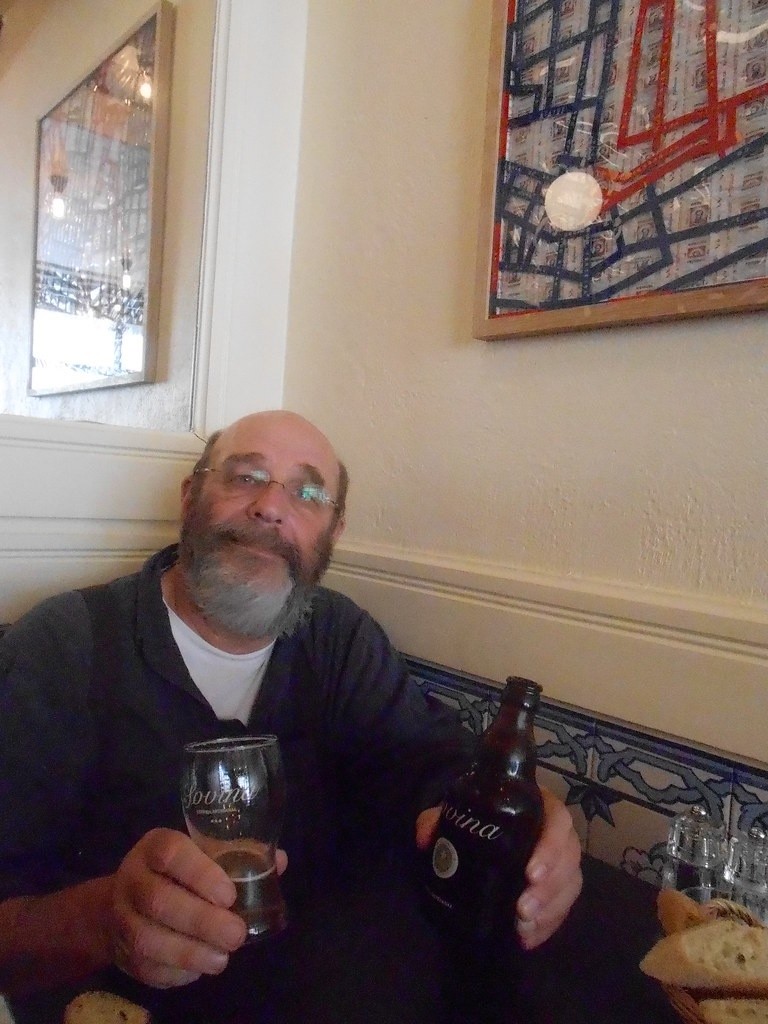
[
  {"xmin": 660, "ymin": 806, "xmax": 728, "ymax": 903},
  {"xmin": 416, "ymin": 676, "xmax": 544, "ymax": 941},
  {"xmin": 724, "ymin": 828, "xmax": 768, "ymax": 925}
]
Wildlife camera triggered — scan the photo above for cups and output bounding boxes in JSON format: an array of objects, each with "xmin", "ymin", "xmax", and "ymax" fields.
[{"xmin": 181, "ymin": 737, "xmax": 285, "ymax": 950}]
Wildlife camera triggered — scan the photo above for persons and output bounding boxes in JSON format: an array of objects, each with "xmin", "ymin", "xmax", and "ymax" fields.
[{"xmin": 0, "ymin": 410, "xmax": 585, "ymax": 1024}]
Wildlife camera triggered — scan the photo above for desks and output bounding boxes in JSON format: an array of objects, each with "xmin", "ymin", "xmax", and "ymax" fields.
[{"xmin": 9, "ymin": 850, "xmax": 682, "ymax": 1024}]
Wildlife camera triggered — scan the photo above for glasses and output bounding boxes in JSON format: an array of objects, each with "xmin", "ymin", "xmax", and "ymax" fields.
[{"xmin": 193, "ymin": 463, "xmax": 343, "ymax": 520}]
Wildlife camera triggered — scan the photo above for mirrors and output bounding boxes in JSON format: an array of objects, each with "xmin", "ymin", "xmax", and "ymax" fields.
[{"xmin": 0, "ymin": 0, "xmax": 233, "ymax": 453}]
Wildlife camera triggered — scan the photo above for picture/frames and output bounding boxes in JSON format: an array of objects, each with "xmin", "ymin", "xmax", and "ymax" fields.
[
  {"xmin": 474, "ymin": 0, "xmax": 768, "ymax": 345},
  {"xmin": 20, "ymin": 0, "xmax": 178, "ymax": 398}
]
